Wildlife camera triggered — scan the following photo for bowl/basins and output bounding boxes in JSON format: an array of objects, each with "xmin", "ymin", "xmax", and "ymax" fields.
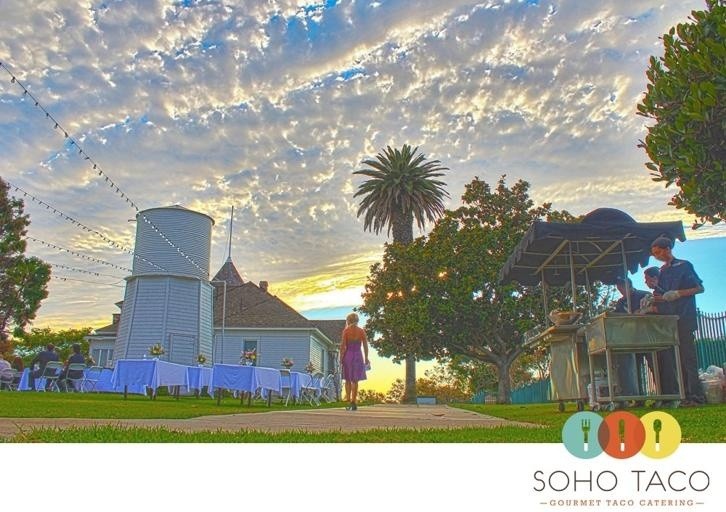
[{"xmin": 547, "ymin": 313, "xmax": 584, "ymax": 325}]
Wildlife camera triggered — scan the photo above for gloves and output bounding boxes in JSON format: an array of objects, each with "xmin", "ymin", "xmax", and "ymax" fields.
[{"xmin": 661, "ymin": 290, "xmax": 681, "ymax": 303}]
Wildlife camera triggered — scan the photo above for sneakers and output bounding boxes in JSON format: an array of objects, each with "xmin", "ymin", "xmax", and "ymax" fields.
[{"xmin": 631, "ymin": 400, "xmax": 706, "ymax": 408}]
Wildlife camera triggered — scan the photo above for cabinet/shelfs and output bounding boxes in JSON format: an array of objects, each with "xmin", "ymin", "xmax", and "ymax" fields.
[{"xmin": 549, "ymin": 311, "xmax": 686, "ymax": 405}]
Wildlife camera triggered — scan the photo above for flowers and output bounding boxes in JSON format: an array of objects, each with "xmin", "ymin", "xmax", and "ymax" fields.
[
  {"xmin": 240, "ymin": 347, "xmax": 259, "ymax": 359},
  {"xmin": 146, "ymin": 341, "xmax": 167, "ymax": 358}
]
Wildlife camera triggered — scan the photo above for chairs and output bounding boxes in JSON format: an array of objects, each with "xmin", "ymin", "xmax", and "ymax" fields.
[{"xmin": 1, "ymin": 359, "xmax": 334, "ymax": 409}]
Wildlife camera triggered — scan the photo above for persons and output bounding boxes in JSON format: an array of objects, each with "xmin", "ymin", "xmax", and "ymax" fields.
[
  {"xmin": 613, "ymin": 237, "xmax": 707, "ymax": 408},
  {"xmin": 340, "ymin": 313, "xmax": 370, "ymax": 410}
]
